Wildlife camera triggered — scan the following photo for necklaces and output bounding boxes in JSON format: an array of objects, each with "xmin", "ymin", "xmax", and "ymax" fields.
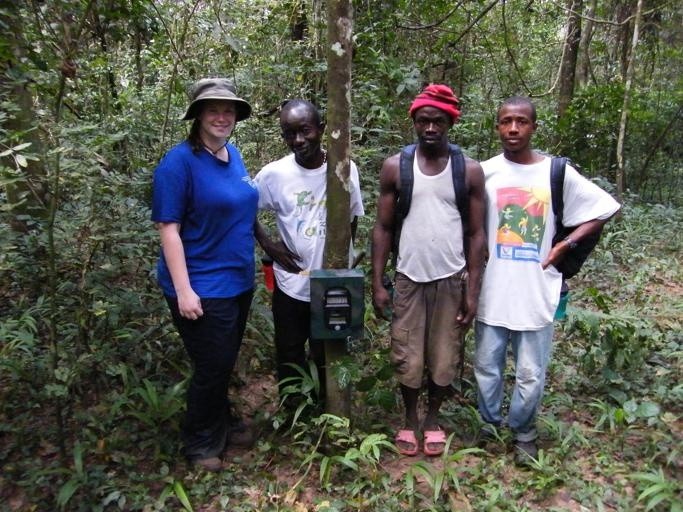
[
  {"xmin": 319, "ymin": 148, "xmax": 330, "ymax": 168},
  {"xmin": 202, "ymin": 142, "xmax": 229, "ymax": 160}
]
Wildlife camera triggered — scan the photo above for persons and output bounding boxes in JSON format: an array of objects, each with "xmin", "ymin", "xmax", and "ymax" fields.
[
  {"xmin": 461, "ymin": 97, "xmax": 623, "ymax": 469},
  {"xmin": 148, "ymin": 77, "xmax": 260, "ymax": 476},
  {"xmin": 370, "ymin": 85, "xmax": 488, "ymax": 458},
  {"xmin": 252, "ymin": 99, "xmax": 366, "ymax": 454}
]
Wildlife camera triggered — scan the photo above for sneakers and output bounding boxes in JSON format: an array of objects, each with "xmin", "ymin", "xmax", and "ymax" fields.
[
  {"xmin": 464, "ymin": 428, "xmax": 500, "ymax": 449},
  {"xmin": 225, "ymin": 424, "xmax": 253, "ymax": 448},
  {"xmin": 512, "ymin": 439, "xmax": 538, "ymax": 471},
  {"xmin": 191, "ymin": 457, "xmax": 222, "ymax": 473}
]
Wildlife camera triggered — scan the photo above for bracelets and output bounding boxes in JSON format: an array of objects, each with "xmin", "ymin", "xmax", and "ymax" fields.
[{"xmin": 561, "ymin": 235, "xmax": 580, "ymax": 251}]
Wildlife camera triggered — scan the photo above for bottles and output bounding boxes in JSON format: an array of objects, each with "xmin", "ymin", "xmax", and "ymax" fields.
[
  {"xmin": 552, "ymin": 282, "xmax": 569, "ymax": 321},
  {"xmin": 261, "ymin": 255, "xmax": 275, "ymax": 294},
  {"xmin": 380, "ymin": 273, "xmax": 394, "ymax": 320}
]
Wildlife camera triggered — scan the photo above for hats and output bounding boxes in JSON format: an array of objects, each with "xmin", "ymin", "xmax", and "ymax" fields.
[
  {"xmin": 181, "ymin": 78, "xmax": 252, "ymax": 122},
  {"xmin": 410, "ymin": 84, "xmax": 461, "ymax": 121}
]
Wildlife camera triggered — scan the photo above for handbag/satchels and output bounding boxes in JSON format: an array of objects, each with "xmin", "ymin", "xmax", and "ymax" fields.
[{"xmin": 552, "ymin": 222, "xmax": 604, "ymax": 279}]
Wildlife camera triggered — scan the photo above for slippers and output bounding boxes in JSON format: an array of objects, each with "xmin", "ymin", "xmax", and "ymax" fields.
[
  {"xmin": 423, "ymin": 424, "xmax": 447, "ymax": 455},
  {"xmin": 395, "ymin": 429, "xmax": 419, "ymax": 455}
]
